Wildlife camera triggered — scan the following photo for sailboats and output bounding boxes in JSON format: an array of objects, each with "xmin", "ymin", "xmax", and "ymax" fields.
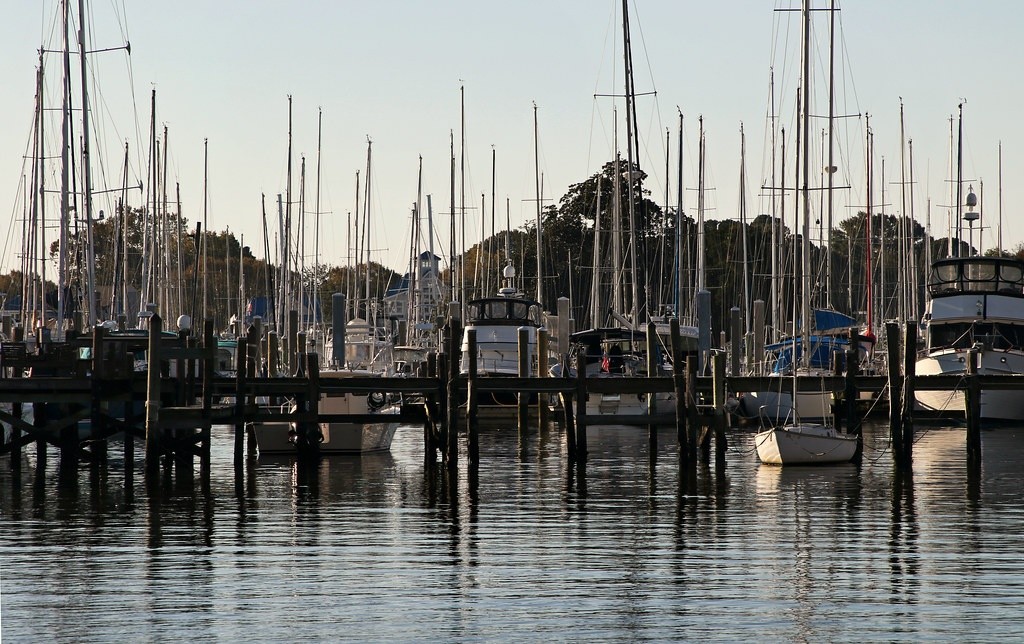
[{"xmin": 1, "ymin": 0, "xmax": 1023, "ymax": 466}]
